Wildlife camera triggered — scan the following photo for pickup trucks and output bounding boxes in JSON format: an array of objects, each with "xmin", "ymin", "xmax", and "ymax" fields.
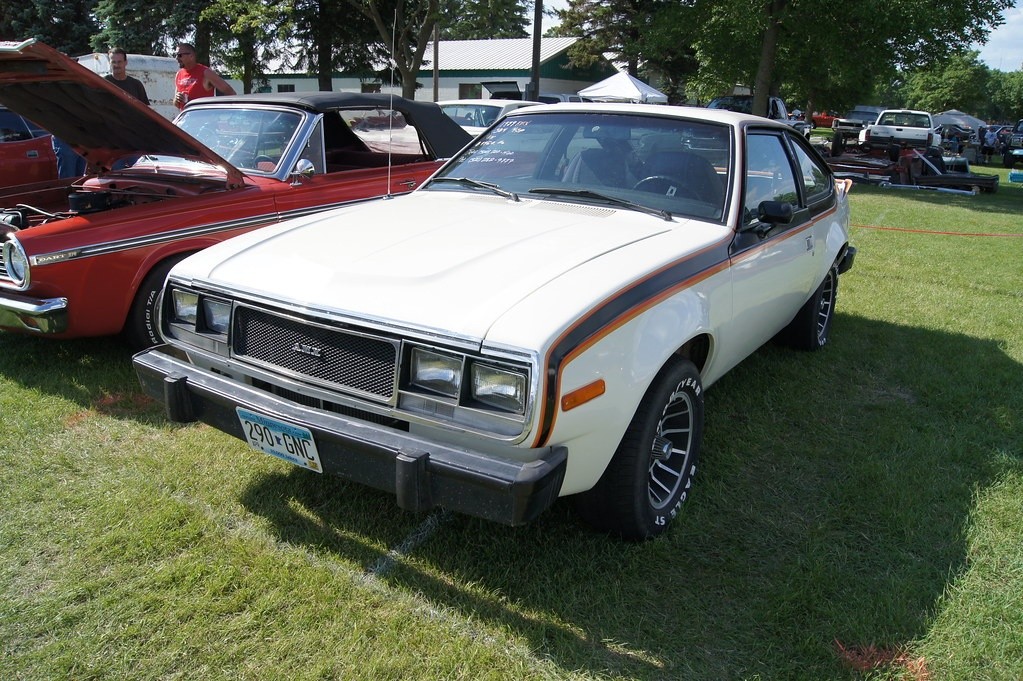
[
  {"xmin": 798, "ymin": 109, "xmax": 837, "ymax": 129},
  {"xmin": 865, "ymin": 110, "xmax": 934, "ymax": 150},
  {"xmin": 1002, "ymin": 119, "xmax": 1023, "ymax": 167},
  {"xmin": 686, "ymin": 95, "xmax": 810, "ymax": 162}
]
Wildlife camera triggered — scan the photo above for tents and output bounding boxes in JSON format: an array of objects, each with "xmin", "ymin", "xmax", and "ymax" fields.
[
  {"xmin": 923, "ymin": 109, "xmax": 986, "ymax": 136},
  {"xmin": 576, "ymin": 71, "xmax": 669, "ymax": 104}
]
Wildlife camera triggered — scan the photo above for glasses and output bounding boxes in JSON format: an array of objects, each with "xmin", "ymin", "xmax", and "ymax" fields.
[{"xmin": 172, "ymin": 52, "xmax": 191, "ymax": 58}]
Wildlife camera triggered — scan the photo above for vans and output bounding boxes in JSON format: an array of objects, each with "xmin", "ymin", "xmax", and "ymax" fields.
[{"xmin": 538, "ymin": 94, "xmax": 596, "ymax": 103}]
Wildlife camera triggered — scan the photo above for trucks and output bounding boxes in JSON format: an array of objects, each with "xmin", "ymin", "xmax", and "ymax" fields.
[{"xmin": 831, "ymin": 106, "xmax": 887, "ymax": 140}]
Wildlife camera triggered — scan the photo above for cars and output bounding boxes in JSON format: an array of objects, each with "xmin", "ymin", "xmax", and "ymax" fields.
[
  {"xmin": 391, "ymin": 98, "xmax": 547, "ymax": 149},
  {"xmin": 994, "ymin": 126, "xmax": 1015, "ymax": 146},
  {"xmin": 933, "ymin": 126, "xmax": 969, "ymax": 141},
  {"xmin": 0, "ymin": 36, "xmax": 508, "ymax": 349},
  {"xmin": 129, "ymin": 102, "xmax": 858, "ymax": 553},
  {"xmin": 0, "ymin": 102, "xmax": 77, "ymax": 199},
  {"xmin": 940, "ymin": 124, "xmax": 975, "ymax": 138},
  {"xmin": 986, "ymin": 125, "xmax": 1001, "ymax": 135}
]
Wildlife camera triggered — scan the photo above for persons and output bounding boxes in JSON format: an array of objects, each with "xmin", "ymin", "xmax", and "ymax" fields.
[
  {"xmin": 103, "ymin": 47, "xmax": 151, "ymax": 170},
  {"xmin": 173, "ymin": 44, "xmax": 236, "ymax": 113},
  {"xmin": 50, "ymin": 52, "xmax": 87, "ymax": 179},
  {"xmin": 981, "ymin": 126, "xmax": 997, "ymax": 162},
  {"xmin": 978, "ymin": 125, "xmax": 987, "ymax": 141},
  {"xmin": 944, "ymin": 125, "xmax": 963, "ymax": 142}
]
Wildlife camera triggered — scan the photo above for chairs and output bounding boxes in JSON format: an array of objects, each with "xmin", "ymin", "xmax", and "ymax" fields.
[
  {"xmin": 563, "ymin": 148, "xmax": 631, "ymax": 190},
  {"xmin": 638, "ymin": 152, "xmax": 726, "ymax": 206}
]
